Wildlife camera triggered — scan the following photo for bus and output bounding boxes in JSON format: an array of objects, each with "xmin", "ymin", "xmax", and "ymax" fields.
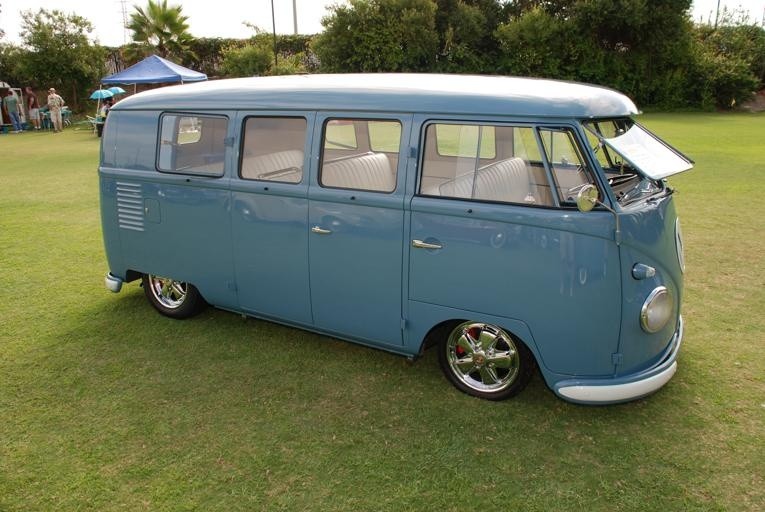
[{"xmin": 96, "ymin": 72, "xmax": 695, "ymax": 406}]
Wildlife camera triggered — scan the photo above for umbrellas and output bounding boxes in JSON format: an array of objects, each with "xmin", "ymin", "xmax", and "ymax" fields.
[
  {"xmin": 89, "ymin": 89, "xmax": 114, "ymax": 100},
  {"xmin": 107, "ymin": 87, "xmax": 126, "ymax": 94}
]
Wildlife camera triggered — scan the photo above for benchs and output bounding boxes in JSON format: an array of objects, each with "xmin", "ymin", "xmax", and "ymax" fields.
[
  {"xmin": 427, "ymin": 154, "xmax": 534, "ymax": 207},
  {"xmin": 180, "ymin": 133, "xmax": 301, "ymax": 226},
  {"xmin": 316, "ymin": 152, "xmax": 398, "ymax": 197}
]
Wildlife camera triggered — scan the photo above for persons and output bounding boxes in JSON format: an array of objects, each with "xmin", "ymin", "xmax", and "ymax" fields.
[
  {"xmin": 47, "ymin": 87, "xmax": 66, "ymax": 133},
  {"xmin": 100, "ymin": 97, "xmax": 116, "ymax": 122},
  {"xmin": 25, "ymin": 86, "xmax": 41, "ymax": 131},
  {"xmin": 3, "ymin": 90, "xmax": 22, "ymax": 133}
]
